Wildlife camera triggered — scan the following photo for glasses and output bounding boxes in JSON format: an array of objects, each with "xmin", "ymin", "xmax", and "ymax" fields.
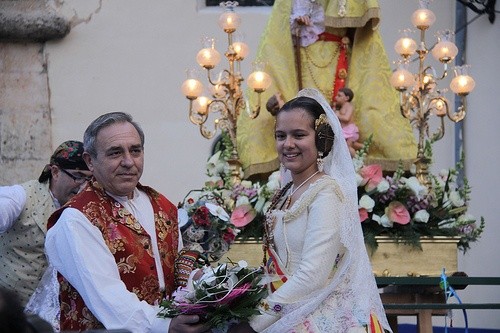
[{"xmin": 57, "ymin": 165, "xmax": 90, "ymax": 184}]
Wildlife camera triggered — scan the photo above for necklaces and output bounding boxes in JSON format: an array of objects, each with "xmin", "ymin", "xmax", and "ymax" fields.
[{"xmin": 288, "ymin": 169, "xmax": 320, "ymax": 196}]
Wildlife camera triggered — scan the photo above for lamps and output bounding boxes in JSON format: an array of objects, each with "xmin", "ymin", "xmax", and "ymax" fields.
[
  {"xmin": 179, "ymin": 0, "xmax": 271, "ymax": 155},
  {"xmin": 389, "ymin": 7, "xmax": 476, "ymax": 143}
]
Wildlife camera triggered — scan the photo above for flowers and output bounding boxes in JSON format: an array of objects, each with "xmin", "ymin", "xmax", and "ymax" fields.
[
  {"xmin": 157, "ymin": 163, "xmax": 279, "ymax": 333},
  {"xmin": 356, "ymin": 164, "xmax": 485, "ymax": 257}
]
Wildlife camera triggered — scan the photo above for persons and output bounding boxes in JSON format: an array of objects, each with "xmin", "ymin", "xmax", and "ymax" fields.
[
  {"xmin": 331, "ymin": 89, "xmax": 368, "ymax": 157},
  {"xmin": 0, "ymin": 140, "xmax": 95, "ymax": 312},
  {"xmin": 266, "ymin": 93, "xmax": 283, "ymax": 115},
  {"xmin": 235, "ymin": 0, "xmax": 418, "ymax": 180},
  {"xmin": 43, "ymin": 112, "xmax": 214, "ymax": 331},
  {"xmin": 226, "ymin": 88, "xmax": 395, "ymax": 333}
]
